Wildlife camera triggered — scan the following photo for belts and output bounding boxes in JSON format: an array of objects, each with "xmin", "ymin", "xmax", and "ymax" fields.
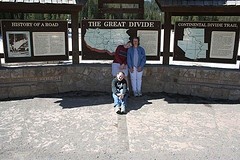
[{"xmin": 133, "ymin": 65, "xmax": 140, "ymax": 67}]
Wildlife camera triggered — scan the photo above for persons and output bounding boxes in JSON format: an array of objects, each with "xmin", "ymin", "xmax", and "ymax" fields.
[
  {"xmin": 111, "ymin": 41, "xmax": 133, "ymax": 91},
  {"xmin": 127, "ymin": 37, "xmax": 147, "ymax": 97},
  {"xmin": 112, "ymin": 72, "xmax": 129, "ymax": 114}
]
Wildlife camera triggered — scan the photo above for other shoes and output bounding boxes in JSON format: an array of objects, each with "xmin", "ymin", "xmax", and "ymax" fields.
[
  {"xmin": 115, "ymin": 107, "xmax": 121, "ymax": 113},
  {"xmin": 134, "ymin": 93, "xmax": 142, "ymax": 97},
  {"xmin": 121, "ymin": 107, "xmax": 126, "ymax": 113}
]
